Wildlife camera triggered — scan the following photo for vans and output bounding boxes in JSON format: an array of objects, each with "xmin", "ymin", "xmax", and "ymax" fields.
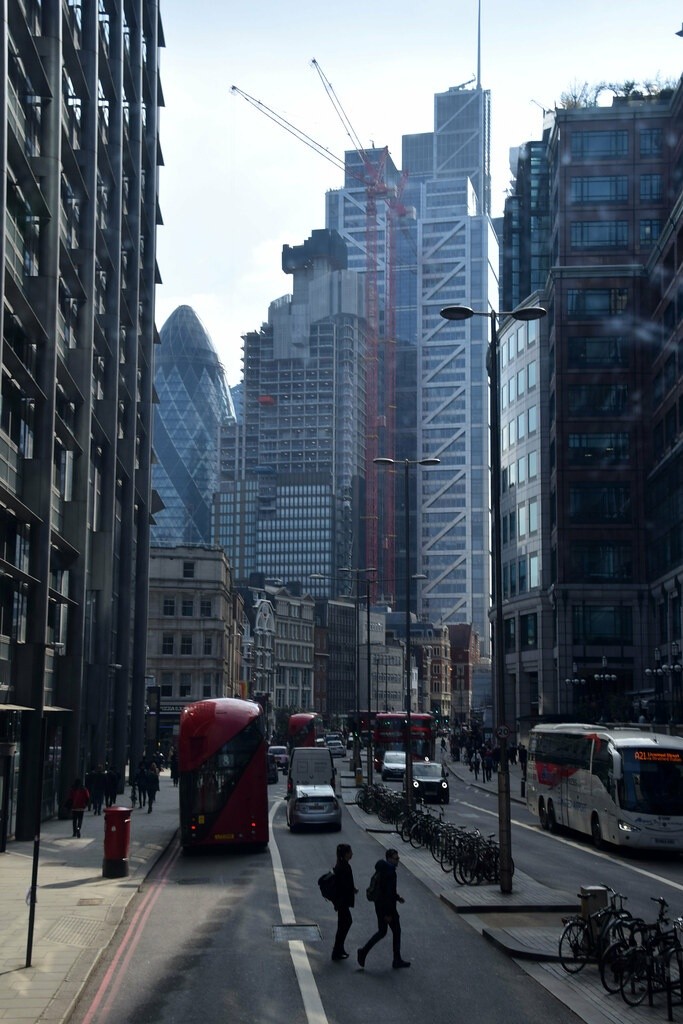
[
  {"xmin": 380, "ymin": 751, "xmax": 406, "ymax": 780},
  {"xmin": 282, "ymin": 747, "xmax": 337, "ymax": 795}
]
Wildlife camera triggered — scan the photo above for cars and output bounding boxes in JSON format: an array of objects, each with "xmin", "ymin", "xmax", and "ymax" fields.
[
  {"xmin": 268, "ymin": 744, "xmax": 289, "ymax": 781},
  {"xmin": 438, "ymin": 725, "xmax": 451, "ymax": 737},
  {"xmin": 326, "ymin": 732, "xmax": 347, "ymax": 756},
  {"xmin": 347, "ymin": 733, "xmax": 366, "ymax": 750},
  {"xmin": 285, "ymin": 783, "xmax": 342, "ymax": 834}
]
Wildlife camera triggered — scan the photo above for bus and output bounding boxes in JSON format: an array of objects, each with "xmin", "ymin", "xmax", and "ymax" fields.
[
  {"xmin": 372, "ymin": 712, "xmax": 437, "ymax": 772},
  {"xmin": 178, "ymin": 697, "xmax": 273, "ymax": 853},
  {"xmin": 289, "ymin": 712, "xmax": 325, "ymax": 749},
  {"xmin": 524, "ymin": 717, "xmax": 683, "ymax": 852}
]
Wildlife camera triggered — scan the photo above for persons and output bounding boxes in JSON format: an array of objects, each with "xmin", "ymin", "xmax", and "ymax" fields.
[
  {"xmin": 88, "ymin": 761, "xmax": 121, "ymax": 816},
  {"xmin": 136, "ymin": 742, "xmax": 179, "ymax": 813},
  {"xmin": 357, "ymin": 848, "xmax": 412, "ymax": 968},
  {"xmin": 441, "ymin": 729, "xmax": 529, "ymax": 784},
  {"xmin": 65, "ymin": 779, "xmax": 90, "ymax": 838},
  {"xmin": 331, "ymin": 843, "xmax": 358, "ymax": 960}
]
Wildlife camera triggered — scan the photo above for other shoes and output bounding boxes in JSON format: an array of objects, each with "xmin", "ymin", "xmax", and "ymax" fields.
[
  {"xmin": 332, "ymin": 948, "xmax": 349, "ymax": 961},
  {"xmin": 393, "ymin": 960, "xmax": 410, "ymax": 969},
  {"xmin": 76, "ymin": 827, "xmax": 80, "ymax": 838},
  {"xmin": 357, "ymin": 949, "xmax": 364, "ymax": 967},
  {"xmin": 73, "ymin": 827, "xmax": 76, "ymax": 836}
]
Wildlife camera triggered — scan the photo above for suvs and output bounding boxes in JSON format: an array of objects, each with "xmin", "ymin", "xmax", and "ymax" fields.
[
  {"xmin": 348, "ymin": 710, "xmax": 389, "ymax": 739},
  {"xmin": 403, "ymin": 762, "xmax": 450, "ymax": 803}
]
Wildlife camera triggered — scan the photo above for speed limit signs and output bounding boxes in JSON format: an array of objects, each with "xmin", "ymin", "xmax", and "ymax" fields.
[{"xmin": 495, "ymin": 724, "xmax": 511, "ymax": 737}]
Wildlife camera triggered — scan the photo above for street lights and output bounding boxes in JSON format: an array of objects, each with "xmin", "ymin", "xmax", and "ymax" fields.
[
  {"xmin": 308, "ymin": 566, "xmax": 428, "ymax": 783},
  {"xmin": 441, "ymin": 304, "xmax": 559, "ymax": 894},
  {"xmin": 147, "ymin": 685, "xmax": 162, "ymax": 769},
  {"xmin": 228, "ymin": 57, "xmax": 409, "ymax": 609},
  {"xmin": 373, "ymin": 456, "xmax": 441, "ymax": 753}
]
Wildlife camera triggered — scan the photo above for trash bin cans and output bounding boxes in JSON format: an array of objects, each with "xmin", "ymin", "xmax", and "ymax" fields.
[
  {"xmin": 580, "ymin": 884, "xmax": 611, "ymax": 953},
  {"xmin": 103, "ymin": 807, "xmax": 133, "ymax": 878},
  {"xmin": 356, "ymin": 768, "xmax": 363, "ymax": 787}
]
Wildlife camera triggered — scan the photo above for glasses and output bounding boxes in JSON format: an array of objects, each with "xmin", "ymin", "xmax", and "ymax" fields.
[{"xmin": 391, "ymin": 857, "xmax": 399, "ymax": 861}]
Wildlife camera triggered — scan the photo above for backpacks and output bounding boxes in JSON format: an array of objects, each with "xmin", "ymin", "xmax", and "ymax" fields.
[
  {"xmin": 366, "ymin": 868, "xmax": 380, "ymax": 901},
  {"xmin": 318, "ymin": 868, "xmax": 337, "ymax": 902}
]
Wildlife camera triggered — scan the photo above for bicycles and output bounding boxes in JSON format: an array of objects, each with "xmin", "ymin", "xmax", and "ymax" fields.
[
  {"xmin": 355, "ymin": 782, "xmax": 518, "ymax": 886},
  {"xmin": 556, "ymin": 881, "xmax": 683, "ymax": 1007}
]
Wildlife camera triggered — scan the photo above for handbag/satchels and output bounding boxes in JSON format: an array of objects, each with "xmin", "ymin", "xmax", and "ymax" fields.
[{"xmin": 65, "ymin": 799, "xmax": 72, "ymax": 810}]
